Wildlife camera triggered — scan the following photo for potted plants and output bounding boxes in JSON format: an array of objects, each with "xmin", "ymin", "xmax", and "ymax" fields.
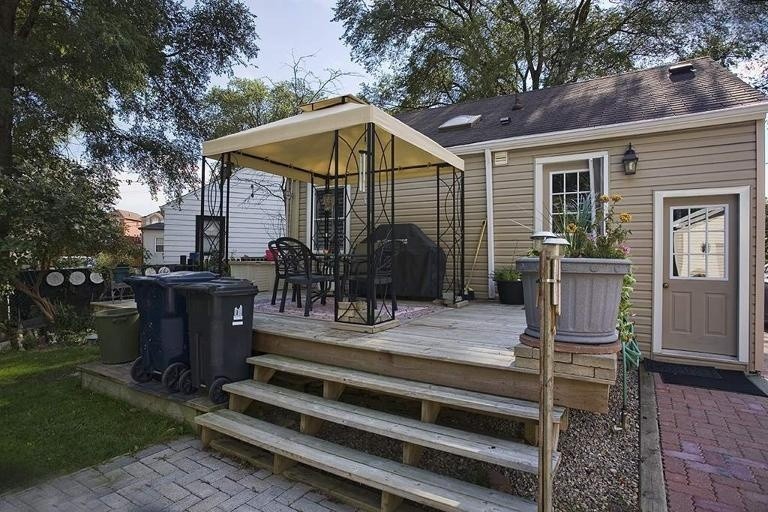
[{"xmin": 490, "ymin": 263, "xmax": 524, "ymax": 305}]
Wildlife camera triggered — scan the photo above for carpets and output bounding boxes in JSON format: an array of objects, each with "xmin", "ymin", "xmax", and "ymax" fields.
[{"xmin": 253, "ymin": 296, "xmax": 455, "ymax": 325}]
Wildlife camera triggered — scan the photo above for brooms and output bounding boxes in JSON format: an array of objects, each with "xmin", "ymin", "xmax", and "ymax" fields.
[{"xmin": 456, "ymin": 221, "xmax": 488, "ymax": 300}]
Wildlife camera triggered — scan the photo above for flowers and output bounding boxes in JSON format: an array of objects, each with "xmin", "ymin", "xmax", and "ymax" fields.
[{"xmin": 527, "ymin": 189, "xmax": 633, "ymax": 259}]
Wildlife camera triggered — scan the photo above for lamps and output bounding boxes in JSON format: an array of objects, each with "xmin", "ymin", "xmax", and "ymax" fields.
[
  {"xmin": 621, "ymin": 142, "xmax": 639, "ymax": 176},
  {"xmin": 322, "ymin": 175, "xmax": 335, "ymax": 212}
]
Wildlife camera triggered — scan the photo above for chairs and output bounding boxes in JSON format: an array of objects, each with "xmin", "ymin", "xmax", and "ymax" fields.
[{"xmin": 268, "ymin": 237, "xmax": 409, "ymax": 317}]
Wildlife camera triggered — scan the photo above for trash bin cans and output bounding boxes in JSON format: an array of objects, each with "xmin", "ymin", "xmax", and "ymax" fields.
[
  {"xmin": 121, "ymin": 270, "xmax": 221, "ymax": 394},
  {"xmin": 177, "ymin": 276, "xmax": 260, "ymax": 405}
]
